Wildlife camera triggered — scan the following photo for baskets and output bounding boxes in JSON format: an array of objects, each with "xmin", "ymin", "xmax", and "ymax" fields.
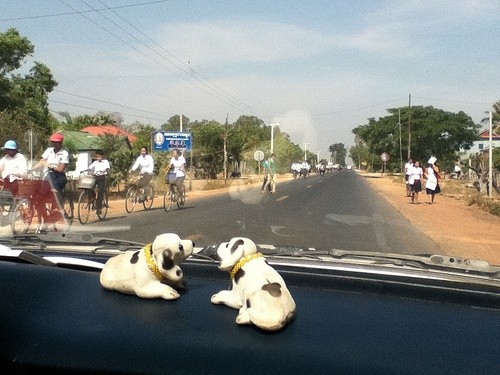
[
  {"xmin": 165, "ymin": 174, "xmax": 177, "ymax": 184},
  {"xmin": 18, "ymin": 180, "xmax": 41, "ymax": 195},
  {"xmin": 79, "ymin": 175, "xmax": 96, "ymax": 190},
  {"xmin": 128, "ymin": 173, "xmax": 145, "ymax": 187}
]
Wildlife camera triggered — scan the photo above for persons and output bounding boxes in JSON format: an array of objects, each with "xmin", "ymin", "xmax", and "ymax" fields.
[
  {"xmin": 424, "ymin": 162, "xmax": 442, "ymax": 204},
  {"xmin": 314, "ymin": 164, "xmax": 336, "ymax": 173},
  {"xmin": 166, "ymin": 149, "xmax": 186, "ymax": 204},
  {"xmin": 405, "ymin": 158, "xmax": 423, "ymax": 204},
  {"xmin": 26, "ymin": 132, "xmax": 69, "ymax": 214},
  {"xmin": 0, "ymin": 140, "xmax": 26, "ymax": 216},
  {"xmin": 455, "ymin": 163, "xmax": 461, "ymax": 177},
  {"xmin": 129, "ymin": 146, "xmax": 154, "ymax": 205},
  {"xmin": 80, "ymin": 149, "xmax": 110, "ymax": 214},
  {"xmin": 291, "ymin": 160, "xmax": 311, "ymax": 178},
  {"xmin": 260, "ymin": 153, "xmax": 276, "ymax": 195}
]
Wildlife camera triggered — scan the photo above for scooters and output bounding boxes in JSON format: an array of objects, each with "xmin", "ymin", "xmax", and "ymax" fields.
[{"xmin": 318, "ymin": 169, "xmax": 324, "ymax": 177}]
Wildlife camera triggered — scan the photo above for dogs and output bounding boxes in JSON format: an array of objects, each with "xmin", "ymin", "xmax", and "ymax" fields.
[
  {"xmin": 100, "ymin": 232, "xmax": 196, "ymax": 300},
  {"xmin": 211, "ymin": 237, "xmax": 298, "ymax": 332}
]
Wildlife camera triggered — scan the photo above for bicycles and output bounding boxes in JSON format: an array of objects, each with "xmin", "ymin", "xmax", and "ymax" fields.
[
  {"xmin": 163, "ymin": 166, "xmax": 187, "ymax": 212},
  {"xmin": 291, "ymin": 167, "xmax": 310, "ymax": 180},
  {"xmin": 0, "ymin": 164, "xmax": 74, "ymax": 238},
  {"xmin": 124, "ymin": 170, "xmax": 155, "ymax": 213},
  {"xmin": 77, "ymin": 170, "xmax": 109, "ymax": 225}
]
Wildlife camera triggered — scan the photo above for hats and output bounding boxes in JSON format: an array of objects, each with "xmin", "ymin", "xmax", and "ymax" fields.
[
  {"xmin": 1, "ymin": 140, "xmax": 18, "ymax": 151},
  {"xmin": 49, "ymin": 133, "xmax": 64, "ymax": 143}
]
